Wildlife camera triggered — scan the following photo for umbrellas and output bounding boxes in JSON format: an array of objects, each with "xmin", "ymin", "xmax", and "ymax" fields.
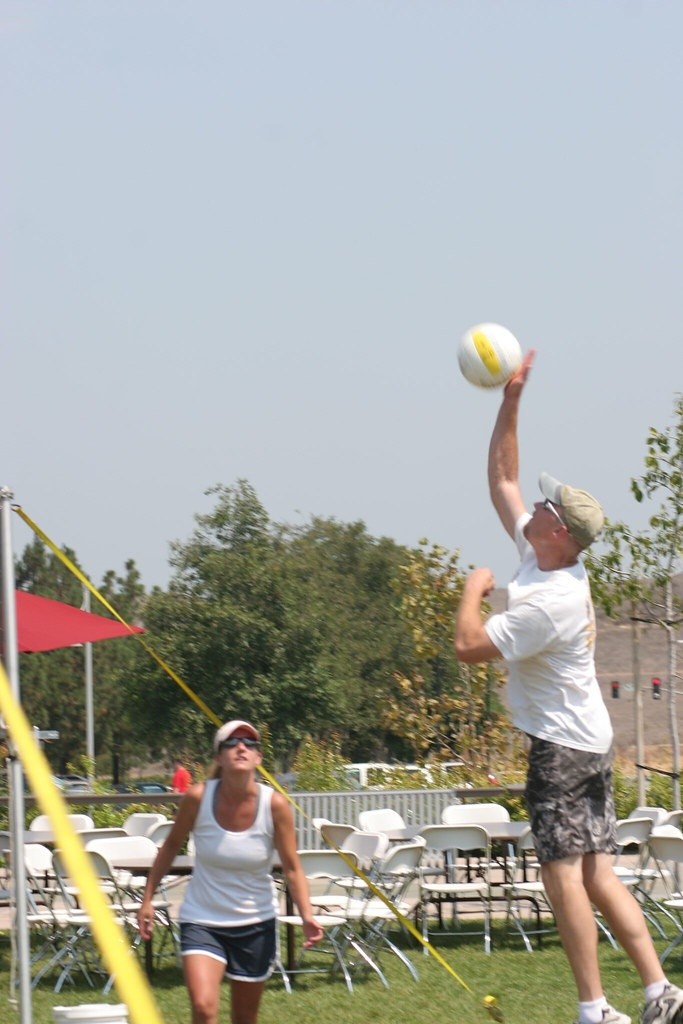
[{"xmin": 0, "ymin": 587, "xmax": 145, "ymax": 656}]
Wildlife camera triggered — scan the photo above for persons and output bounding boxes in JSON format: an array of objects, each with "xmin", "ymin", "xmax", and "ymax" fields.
[
  {"xmin": 136, "ymin": 720, "xmax": 324, "ymax": 1024},
  {"xmin": 171, "ymin": 759, "xmax": 193, "ymax": 794},
  {"xmin": 452, "ymin": 349, "xmax": 683, "ymax": 1024}
]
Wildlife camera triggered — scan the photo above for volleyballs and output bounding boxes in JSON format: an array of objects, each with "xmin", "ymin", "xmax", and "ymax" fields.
[{"xmin": 458, "ymin": 322, "xmax": 524, "ymax": 388}]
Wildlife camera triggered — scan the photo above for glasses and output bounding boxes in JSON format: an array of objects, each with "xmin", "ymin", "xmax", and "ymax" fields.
[
  {"xmin": 219, "ymin": 736, "xmax": 257, "ymax": 748},
  {"xmin": 543, "ymin": 498, "xmax": 574, "ymax": 539}
]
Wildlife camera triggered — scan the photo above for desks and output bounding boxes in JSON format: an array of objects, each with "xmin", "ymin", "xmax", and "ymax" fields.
[
  {"xmin": 23, "ymin": 827, "xmax": 57, "ymax": 888},
  {"xmin": 387, "ymin": 822, "xmax": 543, "ymax": 949},
  {"xmin": 106, "ymin": 855, "xmax": 296, "ymax": 980}
]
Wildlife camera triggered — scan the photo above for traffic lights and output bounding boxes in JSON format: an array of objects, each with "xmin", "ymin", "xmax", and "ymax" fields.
[
  {"xmin": 653, "ymin": 678, "xmax": 660, "ymax": 699},
  {"xmin": 612, "ymin": 681, "xmax": 619, "ymax": 698}
]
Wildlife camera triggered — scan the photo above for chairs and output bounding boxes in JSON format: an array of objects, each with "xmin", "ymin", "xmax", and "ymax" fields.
[{"xmin": 23, "ymin": 805, "xmax": 683, "ymax": 993}]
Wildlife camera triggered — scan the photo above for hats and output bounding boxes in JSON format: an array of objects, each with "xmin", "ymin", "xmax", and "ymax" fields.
[
  {"xmin": 539, "ymin": 473, "xmax": 604, "ymax": 548},
  {"xmin": 213, "ymin": 720, "xmax": 259, "ymax": 753}
]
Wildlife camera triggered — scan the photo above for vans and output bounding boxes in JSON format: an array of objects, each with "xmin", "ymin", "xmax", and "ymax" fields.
[{"xmin": 265, "ymin": 761, "xmax": 527, "ymax": 792}]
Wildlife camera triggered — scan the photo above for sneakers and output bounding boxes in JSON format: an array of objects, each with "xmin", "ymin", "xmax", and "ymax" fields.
[
  {"xmin": 572, "ymin": 1005, "xmax": 632, "ymax": 1024},
  {"xmin": 637, "ymin": 983, "xmax": 683, "ymax": 1024}
]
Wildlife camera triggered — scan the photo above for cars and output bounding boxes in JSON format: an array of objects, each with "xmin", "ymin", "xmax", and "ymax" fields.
[
  {"xmin": 53, "ymin": 773, "xmax": 104, "ymax": 793},
  {"xmin": 118, "ymin": 782, "xmax": 176, "ymax": 794}
]
